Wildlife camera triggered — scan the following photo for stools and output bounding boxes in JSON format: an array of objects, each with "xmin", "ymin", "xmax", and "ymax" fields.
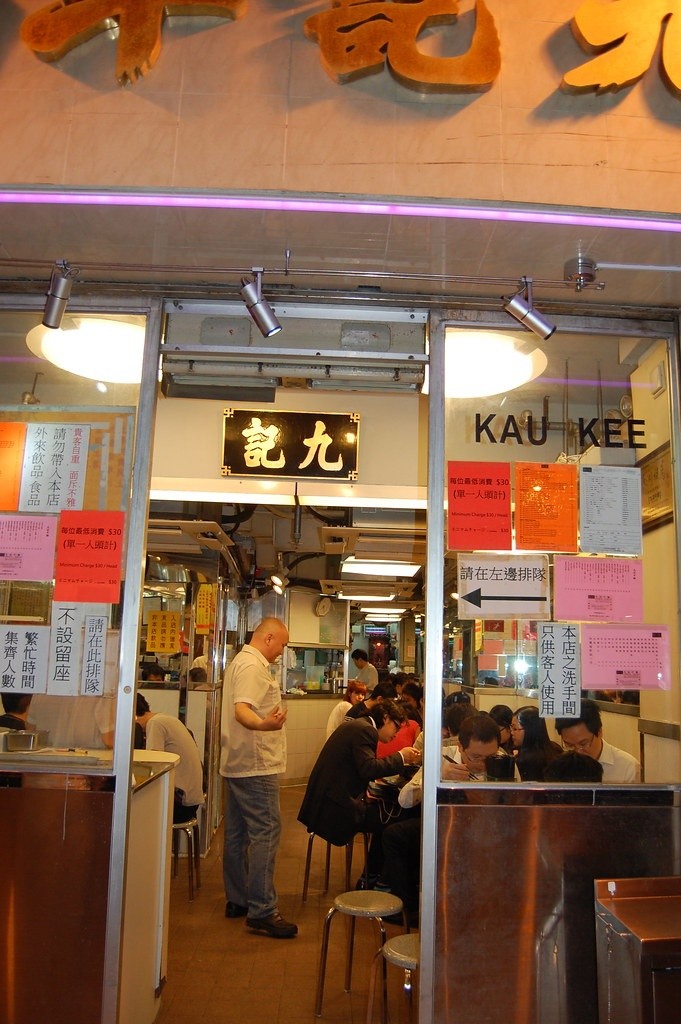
[
  {"xmin": 313, "ymin": 890, "xmax": 413, "ymax": 1024},
  {"xmin": 302, "ymin": 826, "xmax": 369, "ymax": 901},
  {"xmin": 366, "ymin": 933, "xmax": 422, "ymax": 1024},
  {"xmin": 170, "ymin": 816, "xmax": 199, "ymax": 902}
]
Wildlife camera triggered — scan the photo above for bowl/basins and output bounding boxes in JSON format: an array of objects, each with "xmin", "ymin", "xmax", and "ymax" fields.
[{"xmin": 5, "ymin": 730, "xmax": 51, "ymax": 751}]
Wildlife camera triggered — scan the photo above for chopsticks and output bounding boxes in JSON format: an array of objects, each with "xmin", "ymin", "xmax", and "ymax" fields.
[
  {"xmin": 442, "ymin": 753, "xmax": 479, "ymax": 781},
  {"xmin": 484, "ymin": 754, "xmax": 515, "ymax": 778},
  {"xmin": 412, "ymin": 751, "xmax": 417, "ymax": 757}
]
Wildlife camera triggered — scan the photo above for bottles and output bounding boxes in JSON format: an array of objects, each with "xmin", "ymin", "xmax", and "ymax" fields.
[
  {"xmin": 332, "ymin": 679, "xmax": 338, "ymax": 694},
  {"xmin": 164, "ymin": 671, "xmax": 171, "ymax": 682}
]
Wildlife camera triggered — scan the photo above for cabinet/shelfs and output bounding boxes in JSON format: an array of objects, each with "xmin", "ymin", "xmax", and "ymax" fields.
[
  {"xmin": 593, "ymin": 875, "xmax": 681, "ymax": 1024},
  {"xmin": 22, "ymin": 749, "xmax": 180, "ymax": 1024}
]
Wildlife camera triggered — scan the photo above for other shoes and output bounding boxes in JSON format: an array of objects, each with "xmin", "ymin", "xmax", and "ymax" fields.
[
  {"xmin": 225, "ymin": 901, "xmax": 248, "ymax": 917},
  {"xmin": 356, "ymin": 879, "xmax": 376, "ymax": 892},
  {"xmin": 245, "ymin": 911, "xmax": 298, "ymax": 936}
]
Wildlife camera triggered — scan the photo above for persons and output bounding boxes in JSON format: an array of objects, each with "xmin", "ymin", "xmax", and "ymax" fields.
[
  {"xmin": 296, "ymin": 649, "xmax": 641, "ymax": 927},
  {"xmin": 218, "ymin": 617, "xmax": 300, "ymax": 937},
  {"xmin": 0, "ymin": 641, "xmax": 221, "ymax": 821}
]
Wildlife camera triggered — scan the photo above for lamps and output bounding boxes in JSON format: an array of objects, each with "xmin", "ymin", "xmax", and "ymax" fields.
[
  {"xmin": 272, "ymin": 577, "xmax": 289, "ymax": 596},
  {"xmin": 502, "ymin": 276, "xmax": 557, "ymax": 342},
  {"xmin": 270, "ymin": 552, "xmax": 290, "ymax": 587},
  {"xmin": 240, "ymin": 266, "xmax": 283, "ymax": 339},
  {"xmin": 42, "ymin": 259, "xmax": 81, "ymax": 329},
  {"xmin": 336, "ymin": 555, "xmax": 421, "ymax": 622},
  {"xmin": 419, "ymin": 328, "xmax": 549, "ymax": 399}
]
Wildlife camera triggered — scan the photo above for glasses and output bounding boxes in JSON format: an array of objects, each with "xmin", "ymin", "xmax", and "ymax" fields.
[
  {"xmin": 560, "ymin": 734, "xmax": 595, "ymax": 750},
  {"xmin": 508, "ymin": 723, "xmax": 525, "ymax": 734},
  {"xmin": 392, "ymin": 719, "xmax": 403, "ymax": 731},
  {"xmin": 463, "ymin": 749, "xmax": 482, "ymax": 764}
]
